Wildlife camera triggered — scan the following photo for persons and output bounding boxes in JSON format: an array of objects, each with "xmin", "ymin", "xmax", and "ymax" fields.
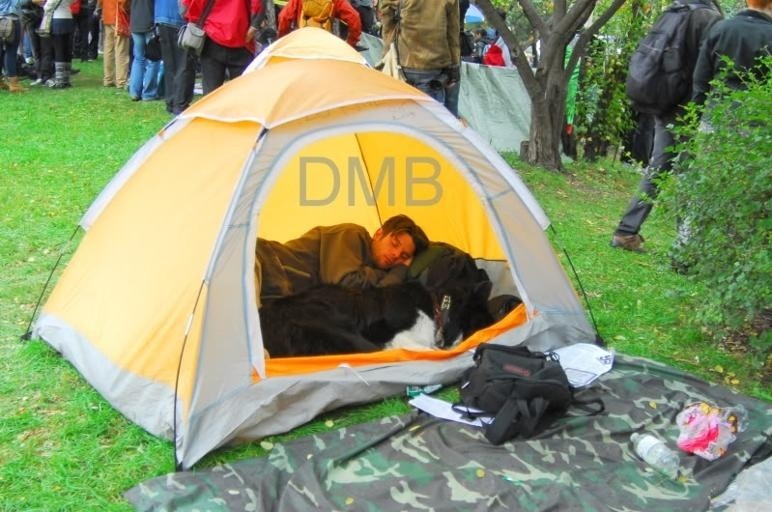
[
  {"xmin": 611, "ymin": 1, "xmax": 725, "ymax": 253},
  {"xmin": 666, "ymin": 1, "xmax": 771, "ymax": 275},
  {"xmin": 0, "ymin": 0, "xmax": 512, "ymax": 117},
  {"xmin": 256, "ymin": 213, "xmax": 430, "ymax": 308}
]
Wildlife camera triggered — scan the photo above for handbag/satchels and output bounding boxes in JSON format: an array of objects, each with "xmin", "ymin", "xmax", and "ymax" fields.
[
  {"xmin": 114, "ymin": 0, "xmax": 133, "ymax": 37},
  {"xmin": 143, "ymin": 34, "xmax": 162, "ymax": 61},
  {"xmin": 176, "ymin": 21, "xmax": 207, "ymax": 58},
  {"xmin": 35, "ymin": 9, "xmax": 54, "ymax": 38}
]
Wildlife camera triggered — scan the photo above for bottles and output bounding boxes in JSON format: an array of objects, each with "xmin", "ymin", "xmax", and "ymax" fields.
[
  {"xmin": 629, "ymin": 429, "xmax": 679, "ymax": 475},
  {"xmin": 432, "ymin": 295, "xmax": 451, "ymax": 341}
]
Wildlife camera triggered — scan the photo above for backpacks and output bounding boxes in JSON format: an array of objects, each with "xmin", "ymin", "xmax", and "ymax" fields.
[
  {"xmin": 624, "ymin": 1, "xmax": 715, "ymax": 116},
  {"xmin": 451, "ymin": 339, "xmax": 606, "ymax": 445}
]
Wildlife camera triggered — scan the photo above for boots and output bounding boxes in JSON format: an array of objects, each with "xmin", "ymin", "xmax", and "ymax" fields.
[
  {"xmin": 0, "ymin": 74, "xmax": 30, "ymax": 92},
  {"xmin": 45, "ymin": 60, "xmax": 71, "ymax": 89}
]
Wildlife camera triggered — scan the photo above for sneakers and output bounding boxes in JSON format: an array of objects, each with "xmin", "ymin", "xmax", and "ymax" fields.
[
  {"xmin": 611, "ymin": 227, "xmax": 653, "ymax": 252},
  {"xmin": 30, "ymin": 78, "xmax": 43, "ymax": 86}
]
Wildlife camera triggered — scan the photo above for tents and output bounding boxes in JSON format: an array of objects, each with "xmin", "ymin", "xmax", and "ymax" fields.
[{"xmin": 22, "ymin": 26, "xmax": 609, "ymax": 473}]
